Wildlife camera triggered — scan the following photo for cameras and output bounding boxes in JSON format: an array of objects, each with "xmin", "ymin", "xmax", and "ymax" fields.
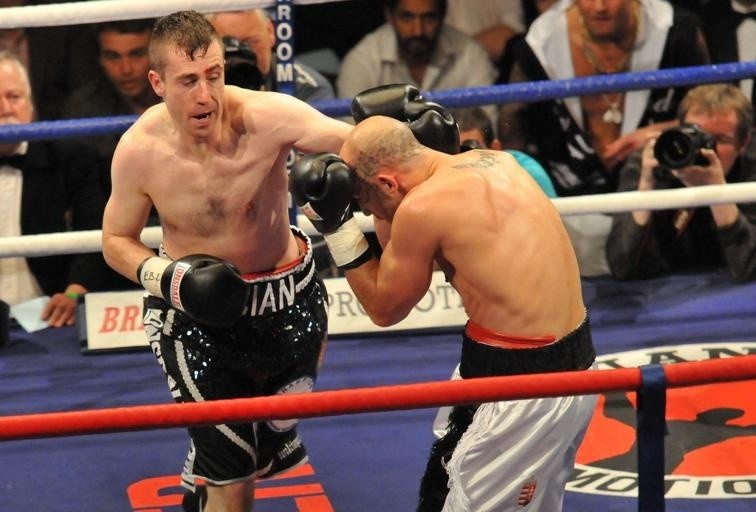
[
  {"xmin": 653, "ymin": 123, "xmax": 715, "ymax": 170},
  {"xmin": 217, "ymin": 37, "xmax": 264, "ymax": 91}
]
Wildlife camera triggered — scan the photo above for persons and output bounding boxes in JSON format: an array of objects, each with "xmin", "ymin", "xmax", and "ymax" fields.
[
  {"xmin": 294, "ymin": 118, "xmax": 602, "ymax": 511},
  {"xmin": 0, "ymin": 0, "xmax": 756, "ymax": 349},
  {"xmin": 99, "ymin": 12, "xmax": 462, "ymax": 512}
]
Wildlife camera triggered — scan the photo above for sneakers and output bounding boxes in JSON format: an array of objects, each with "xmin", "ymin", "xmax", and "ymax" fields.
[{"xmin": 182, "ymin": 484, "xmax": 211, "ymax": 512}]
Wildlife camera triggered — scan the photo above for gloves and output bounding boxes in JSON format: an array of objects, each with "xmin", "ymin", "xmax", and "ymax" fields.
[
  {"xmin": 287, "ymin": 151, "xmax": 374, "ymax": 267},
  {"xmin": 143, "ymin": 253, "xmax": 248, "ymax": 327},
  {"xmin": 348, "ymin": 79, "xmax": 425, "ymax": 133},
  {"xmin": 396, "ymin": 98, "xmax": 462, "ymax": 156}
]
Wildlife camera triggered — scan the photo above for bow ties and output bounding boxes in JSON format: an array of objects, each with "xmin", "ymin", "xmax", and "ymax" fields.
[{"xmin": 0, "ymin": 151, "xmax": 26, "ymax": 173}]
